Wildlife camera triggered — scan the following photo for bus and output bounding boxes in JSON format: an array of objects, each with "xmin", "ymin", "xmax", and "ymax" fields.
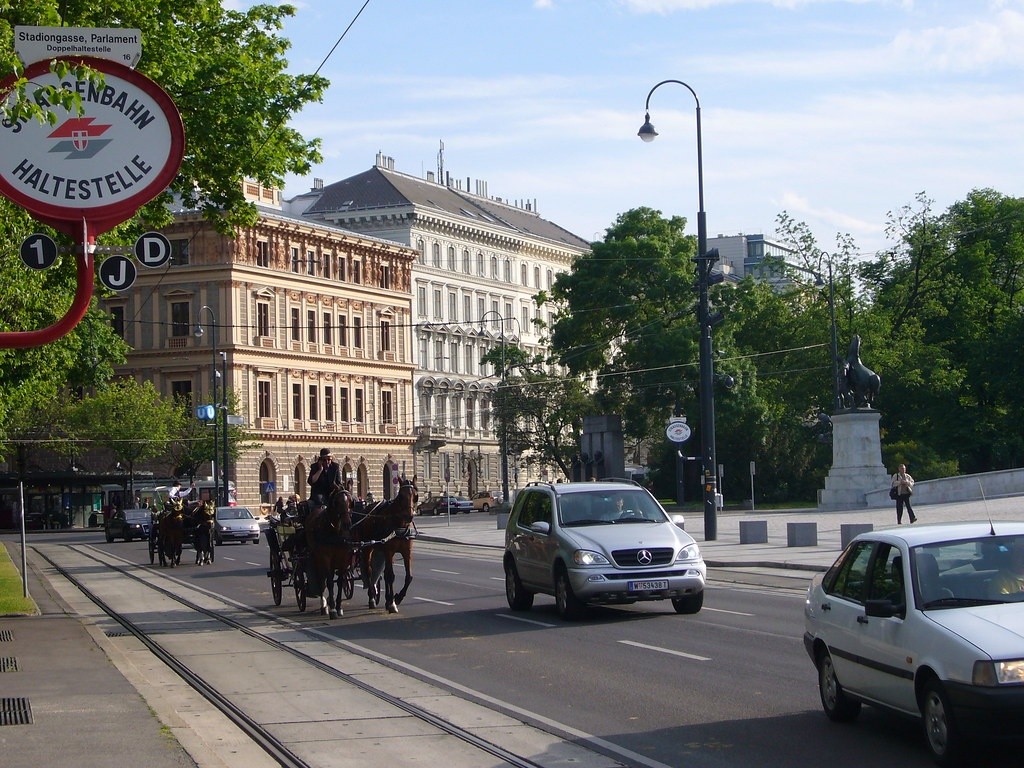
[{"xmin": 101, "ymin": 476, "xmax": 238, "ymax": 528}]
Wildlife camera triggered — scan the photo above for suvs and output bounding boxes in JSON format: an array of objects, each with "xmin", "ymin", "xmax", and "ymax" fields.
[
  {"xmin": 501, "ymin": 476, "xmax": 708, "ymax": 622},
  {"xmin": 471, "ymin": 489, "xmax": 495, "ymax": 512}
]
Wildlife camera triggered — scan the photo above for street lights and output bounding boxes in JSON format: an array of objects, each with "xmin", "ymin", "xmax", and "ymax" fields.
[
  {"xmin": 477, "ymin": 311, "xmax": 513, "ymax": 513},
  {"xmin": 637, "ymin": 78, "xmax": 724, "ymax": 541},
  {"xmin": 194, "ymin": 304, "xmax": 221, "ymax": 506},
  {"xmin": 814, "ymin": 250, "xmax": 844, "ymax": 409}
]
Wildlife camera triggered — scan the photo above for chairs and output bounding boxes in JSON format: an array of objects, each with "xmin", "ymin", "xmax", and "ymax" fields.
[
  {"xmin": 892, "ymin": 554, "xmax": 942, "ymax": 615},
  {"xmin": 564, "ymin": 501, "xmax": 588, "ymax": 524}
]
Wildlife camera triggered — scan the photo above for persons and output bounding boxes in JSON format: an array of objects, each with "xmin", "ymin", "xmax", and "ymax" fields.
[
  {"xmin": 991, "ymin": 541, "xmax": 1024, "ymax": 594},
  {"xmin": 891, "ymin": 465, "xmax": 917, "ymax": 524},
  {"xmin": 110, "ymin": 479, "xmax": 196, "ymax": 519},
  {"xmin": 601, "ymin": 494, "xmax": 642, "ymax": 522},
  {"xmin": 236, "ymin": 511, "xmax": 246, "ymax": 518},
  {"xmin": 548, "ymin": 474, "xmax": 599, "ymax": 484},
  {"xmin": 571, "ymin": 451, "xmax": 606, "ymax": 482},
  {"xmin": 273, "ymin": 448, "xmax": 343, "ymax": 518}
]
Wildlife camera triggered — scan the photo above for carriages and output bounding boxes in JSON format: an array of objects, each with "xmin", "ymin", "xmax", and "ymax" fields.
[
  {"xmin": 259, "ymin": 474, "xmax": 420, "ymax": 619},
  {"xmin": 146, "ymin": 493, "xmax": 219, "ymax": 568}
]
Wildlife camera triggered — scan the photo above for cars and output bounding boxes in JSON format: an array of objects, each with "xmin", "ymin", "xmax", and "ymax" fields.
[
  {"xmin": 801, "ymin": 521, "xmax": 1023, "ymax": 768},
  {"xmin": 455, "ymin": 496, "xmax": 475, "ymax": 514},
  {"xmin": 214, "ymin": 506, "xmax": 261, "ymax": 545},
  {"xmin": 416, "ymin": 495, "xmax": 460, "ymax": 516},
  {"xmin": 105, "ymin": 508, "xmax": 154, "ymax": 544}
]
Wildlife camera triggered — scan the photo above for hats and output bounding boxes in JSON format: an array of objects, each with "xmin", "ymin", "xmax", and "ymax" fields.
[
  {"xmin": 319, "ymin": 448, "xmax": 332, "ymax": 459},
  {"xmin": 173, "ymin": 480, "xmax": 181, "ymax": 486}
]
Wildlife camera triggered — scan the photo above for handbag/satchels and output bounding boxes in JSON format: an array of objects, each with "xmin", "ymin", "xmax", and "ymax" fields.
[{"xmin": 889, "ymin": 488, "xmax": 899, "ymax": 500}]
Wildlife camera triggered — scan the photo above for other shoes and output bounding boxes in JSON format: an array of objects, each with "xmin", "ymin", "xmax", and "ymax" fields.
[
  {"xmin": 910, "ymin": 518, "xmax": 917, "ymax": 524},
  {"xmin": 898, "ymin": 520, "xmax": 902, "ymax": 524}
]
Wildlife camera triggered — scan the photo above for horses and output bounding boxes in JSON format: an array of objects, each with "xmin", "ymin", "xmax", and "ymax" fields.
[
  {"xmin": 158, "ymin": 497, "xmax": 216, "ymax": 568},
  {"xmin": 304, "ymin": 475, "xmax": 418, "ymax": 621},
  {"xmin": 845, "ymin": 334, "xmax": 880, "ymax": 409}
]
